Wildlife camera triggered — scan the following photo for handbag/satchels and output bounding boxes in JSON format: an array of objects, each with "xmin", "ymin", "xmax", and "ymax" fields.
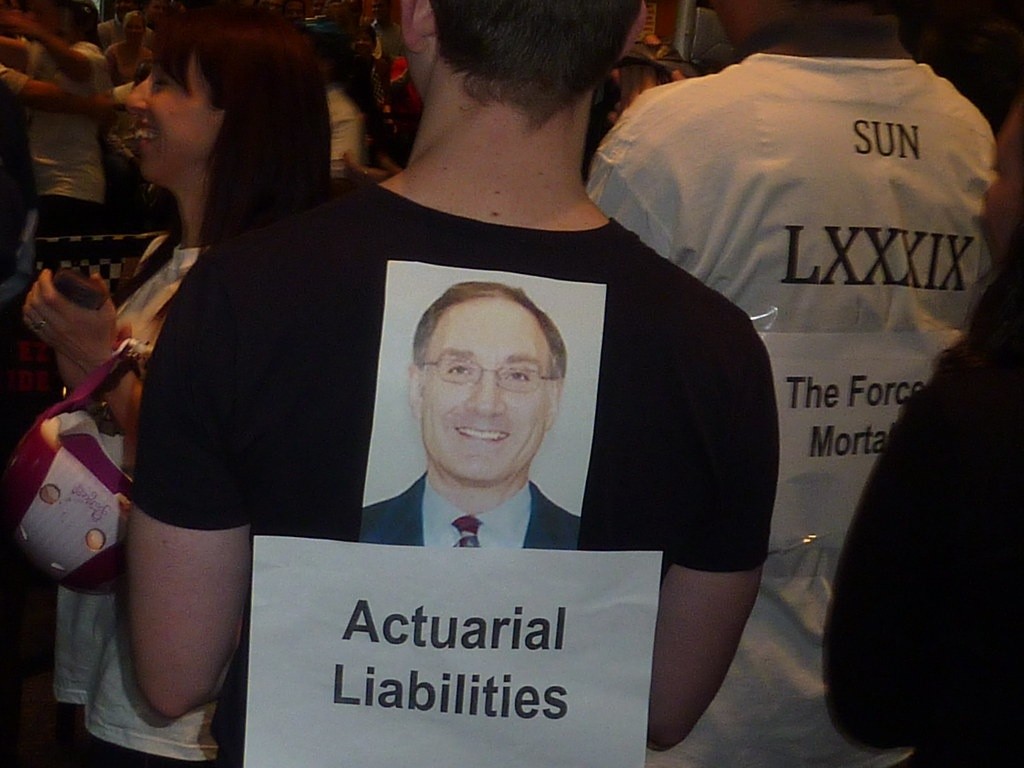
[{"xmin": 2, "ymin": 336, "xmax": 142, "ymax": 592}]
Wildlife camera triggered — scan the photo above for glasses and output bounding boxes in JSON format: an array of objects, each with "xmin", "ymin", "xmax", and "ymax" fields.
[{"xmin": 422, "ymin": 357, "xmax": 560, "ymax": 392}]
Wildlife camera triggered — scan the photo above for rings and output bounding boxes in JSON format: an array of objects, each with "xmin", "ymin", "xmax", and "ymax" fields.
[
  {"xmin": 363, "ymin": 166, "xmax": 369, "ymax": 180},
  {"xmin": 34, "ymin": 319, "xmax": 46, "ymax": 330}
]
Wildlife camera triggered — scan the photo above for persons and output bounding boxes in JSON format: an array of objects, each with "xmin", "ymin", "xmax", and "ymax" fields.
[
  {"xmin": 0, "ymin": 0, "xmax": 1024, "ymax": 768},
  {"xmin": 360, "ymin": 281, "xmax": 581, "ymax": 550}
]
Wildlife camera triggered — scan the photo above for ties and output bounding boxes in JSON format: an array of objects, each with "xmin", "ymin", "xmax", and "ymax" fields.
[{"xmin": 452, "ymin": 515, "xmax": 482, "ymax": 548}]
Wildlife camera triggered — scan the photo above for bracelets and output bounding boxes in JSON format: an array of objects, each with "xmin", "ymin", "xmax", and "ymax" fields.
[{"xmin": 91, "ymin": 358, "xmax": 134, "ymax": 399}]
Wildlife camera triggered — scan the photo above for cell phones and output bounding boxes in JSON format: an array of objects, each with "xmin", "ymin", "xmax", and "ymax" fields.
[{"xmin": 53, "ymin": 270, "xmax": 108, "ymax": 310}]
